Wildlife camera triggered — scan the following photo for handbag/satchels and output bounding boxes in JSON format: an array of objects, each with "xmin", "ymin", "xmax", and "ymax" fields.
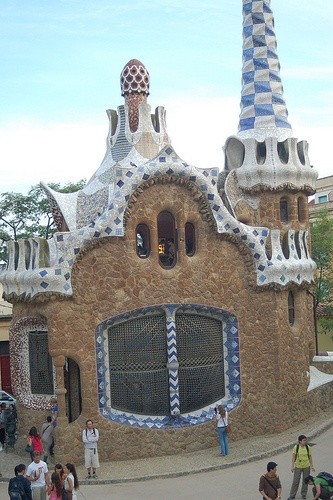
[
  {"xmin": 225, "ymin": 425, "xmax": 231, "ymax": 433},
  {"xmin": 25, "ymin": 440, "xmax": 33, "ymax": 453}
]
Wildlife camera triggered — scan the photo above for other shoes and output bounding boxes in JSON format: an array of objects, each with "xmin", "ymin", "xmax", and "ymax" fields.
[
  {"xmin": 0, "ymin": 447, "xmax": 4, "ymax": 451},
  {"xmin": 87, "ymin": 473, "xmax": 98, "ymax": 479}
]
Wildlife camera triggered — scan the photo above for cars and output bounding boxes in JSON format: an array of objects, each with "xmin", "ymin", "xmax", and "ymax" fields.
[{"xmin": 0, "ymin": 390, "xmax": 17, "ymax": 411}]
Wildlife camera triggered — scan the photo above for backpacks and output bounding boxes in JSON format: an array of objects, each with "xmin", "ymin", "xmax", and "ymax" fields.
[
  {"xmin": 9, "ymin": 476, "xmax": 27, "ymax": 500},
  {"xmin": 315, "ymin": 472, "xmax": 333, "ymax": 487}
]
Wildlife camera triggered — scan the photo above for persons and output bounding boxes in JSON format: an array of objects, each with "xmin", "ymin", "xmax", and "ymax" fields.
[
  {"xmin": 82, "ymin": 420, "xmax": 100, "ymax": 479},
  {"xmin": 48, "ymin": 472, "xmax": 63, "ymax": 500},
  {"xmin": 6, "ymin": 404, "xmax": 18, "ymax": 448},
  {"xmin": 0, "ymin": 403, "xmax": 7, "ymax": 448},
  {"xmin": 167, "ymin": 238, "xmax": 175, "ymax": 265},
  {"xmin": 288, "ymin": 435, "xmax": 315, "ymax": 500},
  {"xmin": 50, "ymin": 398, "xmax": 58, "ymax": 422},
  {"xmin": 216, "ymin": 405, "xmax": 228, "ymax": 456},
  {"xmin": 304, "ymin": 475, "xmax": 333, "ymax": 500},
  {"xmin": 26, "ymin": 451, "xmax": 51, "ymax": 500},
  {"xmin": 64, "ymin": 463, "xmax": 79, "ymax": 500},
  {"xmin": 27, "ymin": 427, "xmax": 44, "ymax": 461},
  {"xmin": 8, "ymin": 464, "xmax": 32, "ymax": 500},
  {"xmin": 55, "ymin": 464, "xmax": 64, "ymax": 479},
  {"xmin": 41, "ymin": 416, "xmax": 54, "ymax": 464},
  {"xmin": 259, "ymin": 462, "xmax": 281, "ymax": 500}
]
